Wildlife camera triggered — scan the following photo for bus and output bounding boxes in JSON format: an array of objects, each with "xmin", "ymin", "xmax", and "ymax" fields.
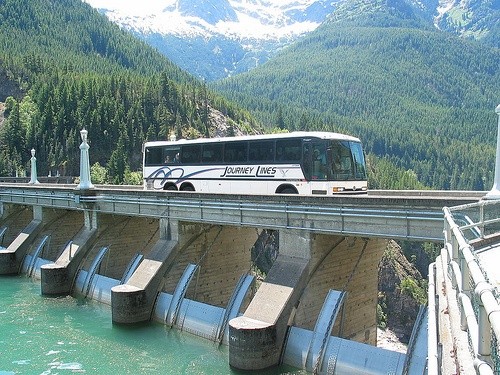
[{"xmin": 144, "ymin": 130, "xmax": 371, "ymax": 199}]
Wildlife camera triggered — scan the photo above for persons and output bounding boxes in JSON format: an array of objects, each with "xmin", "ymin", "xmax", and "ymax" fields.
[{"xmin": 164, "ymin": 146, "xmax": 344, "ymax": 180}]
